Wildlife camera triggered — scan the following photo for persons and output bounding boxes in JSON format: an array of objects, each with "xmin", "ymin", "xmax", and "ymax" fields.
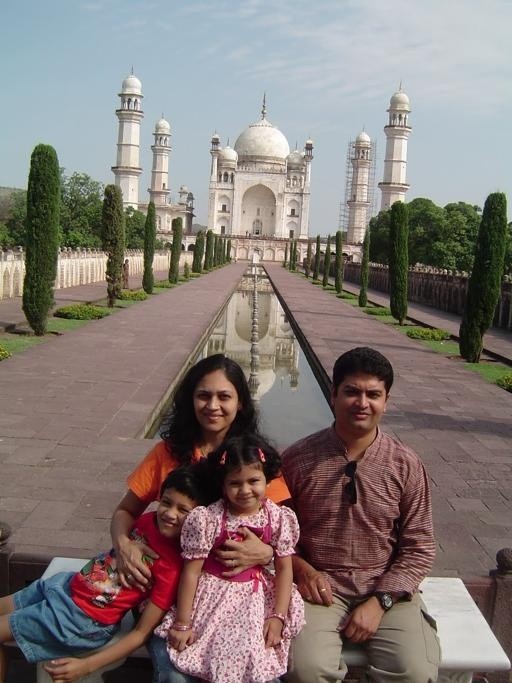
[
  {"xmin": 277, "ymin": 344, "xmax": 442, "ymax": 682},
  {"xmin": 150, "ymin": 434, "xmax": 309, "ymax": 682},
  {"xmin": 109, "ymin": 352, "xmax": 294, "ymax": 683},
  {"xmin": 0, "ymin": 466, "xmax": 215, "ymax": 681}
]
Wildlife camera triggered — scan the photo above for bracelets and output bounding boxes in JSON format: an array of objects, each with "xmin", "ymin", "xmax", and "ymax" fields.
[
  {"xmin": 266, "ymin": 610, "xmax": 289, "ymax": 629},
  {"xmin": 172, "ymin": 624, "xmax": 193, "ymax": 633}
]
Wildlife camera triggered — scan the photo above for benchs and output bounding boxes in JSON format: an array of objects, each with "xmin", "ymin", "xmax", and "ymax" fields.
[{"xmin": 4, "ymin": 555, "xmax": 512, "ymax": 683}]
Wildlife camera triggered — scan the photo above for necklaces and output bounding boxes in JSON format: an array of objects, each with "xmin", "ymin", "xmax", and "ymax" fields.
[
  {"xmin": 202, "ymin": 445, "xmax": 209, "ymax": 460},
  {"xmin": 225, "ymin": 504, "xmax": 266, "ymax": 541}
]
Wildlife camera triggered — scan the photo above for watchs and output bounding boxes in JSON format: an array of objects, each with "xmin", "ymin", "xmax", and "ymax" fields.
[{"xmin": 373, "ymin": 592, "xmax": 395, "ymax": 611}]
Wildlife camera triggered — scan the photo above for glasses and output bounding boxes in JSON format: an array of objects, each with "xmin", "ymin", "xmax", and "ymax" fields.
[{"xmin": 343, "ymin": 459, "xmax": 359, "ymax": 506}]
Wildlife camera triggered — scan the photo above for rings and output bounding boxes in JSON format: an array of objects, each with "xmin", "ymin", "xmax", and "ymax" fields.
[
  {"xmin": 306, "ymin": 594, "xmax": 313, "ymax": 599},
  {"xmin": 127, "ymin": 574, "xmax": 133, "ymax": 580},
  {"xmin": 318, "ymin": 587, "xmax": 326, "ymax": 592}
]
